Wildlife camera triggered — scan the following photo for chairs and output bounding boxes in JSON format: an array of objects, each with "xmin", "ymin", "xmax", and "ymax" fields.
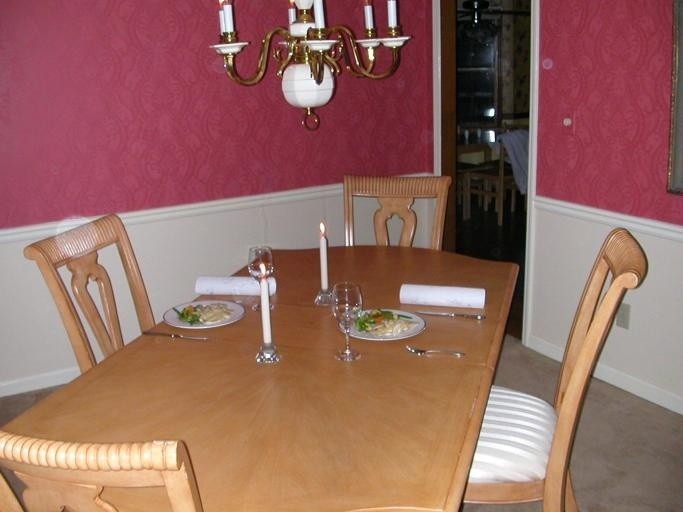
[
  {"xmin": 1, "ymin": 428, "xmax": 204, "ymax": 512},
  {"xmin": 342, "ymin": 173, "xmax": 452, "ymax": 250},
  {"xmin": 24, "ymin": 214, "xmax": 154, "ymax": 372},
  {"xmin": 455, "ymin": 129, "xmax": 527, "ymax": 228},
  {"xmin": 463, "ymin": 227, "xmax": 648, "ymax": 512}
]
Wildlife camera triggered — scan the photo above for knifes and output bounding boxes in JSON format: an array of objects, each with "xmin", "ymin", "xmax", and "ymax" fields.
[
  {"xmin": 413, "ymin": 310, "xmax": 487, "ymax": 321},
  {"xmin": 142, "ymin": 328, "xmax": 209, "ymax": 342}
]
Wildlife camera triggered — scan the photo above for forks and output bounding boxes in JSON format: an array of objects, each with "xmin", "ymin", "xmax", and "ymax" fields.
[{"xmin": 404, "ymin": 343, "xmax": 466, "ymax": 359}]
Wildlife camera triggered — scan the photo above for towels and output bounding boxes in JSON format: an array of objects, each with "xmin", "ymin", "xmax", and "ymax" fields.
[
  {"xmin": 399, "ymin": 283, "xmax": 487, "ymax": 309},
  {"xmin": 194, "ymin": 275, "xmax": 277, "ymax": 298}
]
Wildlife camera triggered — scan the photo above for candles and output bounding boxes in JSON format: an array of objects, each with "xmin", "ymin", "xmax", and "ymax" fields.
[
  {"xmin": 319, "ymin": 221, "xmax": 329, "ymax": 290},
  {"xmin": 260, "ymin": 262, "xmax": 271, "ymax": 344}
]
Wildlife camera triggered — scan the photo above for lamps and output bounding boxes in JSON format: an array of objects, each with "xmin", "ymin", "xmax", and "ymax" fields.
[{"xmin": 207, "ymin": 0, "xmax": 412, "ymax": 132}]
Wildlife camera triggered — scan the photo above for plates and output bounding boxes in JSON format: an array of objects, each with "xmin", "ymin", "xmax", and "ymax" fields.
[
  {"xmin": 162, "ymin": 300, "xmax": 245, "ymax": 330},
  {"xmin": 336, "ymin": 308, "xmax": 427, "ymax": 342}
]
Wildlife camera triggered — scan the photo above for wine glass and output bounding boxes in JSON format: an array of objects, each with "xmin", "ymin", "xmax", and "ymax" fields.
[
  {"xmin": 330, "ymin": 281, "xmax": 363, "ymax": 362},
  {"xmin": 248, "ymin": 245, "xmax": 277, "ymax": 313}
]
